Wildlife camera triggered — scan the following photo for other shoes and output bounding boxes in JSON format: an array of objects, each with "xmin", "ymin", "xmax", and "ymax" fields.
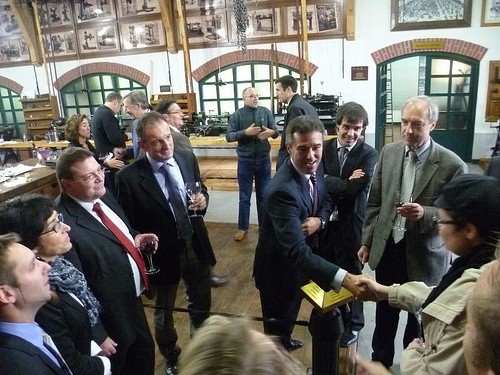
[{"xmin": 234, "ymin": 230, "xmax": 247, "ymax": 240}]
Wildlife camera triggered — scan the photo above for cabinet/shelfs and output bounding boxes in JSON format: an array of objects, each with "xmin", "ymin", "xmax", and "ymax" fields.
[
  {"xmin": 150, "ymin": 93, "xmax": 196, "ymax": 122},
  {"xmin": 19, "ymin": 96, "xmax": 60, "ymax": 140}
]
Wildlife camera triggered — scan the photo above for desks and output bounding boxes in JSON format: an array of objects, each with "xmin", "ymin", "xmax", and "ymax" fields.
[
  {"xmin": 0, "ymin": 140, "xmax": 69, "ymax": 159},
  {"xmin": 190, "ymin": 133, "xmax": 338, "ymax": 149}
]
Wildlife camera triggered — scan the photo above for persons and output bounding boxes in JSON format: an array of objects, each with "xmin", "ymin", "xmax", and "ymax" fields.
[
  {"xmin": 115, "ymin": 113, "xmax": 218, "ymax": 375},
  {"xmin": 226, "ymin": 87, "xmax": 279, "ymax": 241},
  {"xmin": 463, "ymin": 258, "xmax": 500, "ymax": 375},
  {"xmin": 54, "ymin": 147, "xmax": 159, "ymax": 375},
  {"xmin": 155, "ymin": 100, "xmax": 230, "ymax": 286},
  {"xmin": 251, "ymin": 115, "xmax": 379, "ymax": 350},
  {"xmin": 357, "ymin": 94, "xmax": 468, "ymax": 370},
  {"xmin": 275, "ymin": 74, "xmax": 318, "ymax": 171},
  {"xmin": 323, "ymin": 102, "xmax": 378, "ymax": 345},
  {"xmin": 0, "ymin": 192, "xmax": 112, "ymax": 375},
  {"xmin": 177, "ymin": 314, "xmax": 305, "ymax": 375},
  {"xmin": 354, "ymin": 174, "xmax": 500, "ymax": 375},
  {"xmin": 64, "ymin": 91, "xmax": 151, "ymax": 189}
]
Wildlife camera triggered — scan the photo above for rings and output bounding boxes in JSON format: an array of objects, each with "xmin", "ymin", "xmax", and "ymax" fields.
[{"xmin": 405, "ymin": 210, "xmax": 408, "ymax": 213}]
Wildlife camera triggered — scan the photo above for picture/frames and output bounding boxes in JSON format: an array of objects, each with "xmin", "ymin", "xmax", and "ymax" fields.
[
  {"xmin": 390, "ymin": 0, "xmax": 473, "ymax": 31},
  {"xmin": 0, "ymin": 0, "xmax": 348, "ymax": 68},
  {"xmin": 481, "ymin": 0, "xmax": 500, "ymax": 27}
]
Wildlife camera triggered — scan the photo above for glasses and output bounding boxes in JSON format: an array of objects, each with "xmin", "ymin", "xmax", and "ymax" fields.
[
  {"xmin": 432, "ymin": 216, "xmax": 459, "ymax": 226},
  {"xmin": 39, "ymin": 213, "xmax": 64, "ymax": 237},
  {"xmin": 246, "ymin": 94, "xmax": 260, "ymax": 99},
  {"xmin": 72, "ymin": 167, "xmax": 105, "ymax": 182}
]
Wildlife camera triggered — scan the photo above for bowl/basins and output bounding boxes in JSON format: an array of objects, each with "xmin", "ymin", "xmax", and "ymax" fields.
[{"xmin": 53, "ymin": 118, "xmax": 66, "ymax": 125}]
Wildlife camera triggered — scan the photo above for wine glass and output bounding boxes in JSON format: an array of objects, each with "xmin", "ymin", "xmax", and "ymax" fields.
[
  {"xmin": 392, "ymin": 190, "xmax": 408, "ymax": 232},
  {"xmin": 412, "ymin": 295, "xmax": 426, "ymax": 348},
  {"xmin": 185, "ymin": 181, "xmax": 203, "ymax": 217},
  {"xmin": 140, "ymin": 233, "xmax": 160, "ymax": 274}
]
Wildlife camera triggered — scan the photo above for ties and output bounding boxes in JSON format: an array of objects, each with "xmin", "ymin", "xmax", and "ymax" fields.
[
  {"xmin": 159, "ymin": 165, "xmax": 193, "ymax": 240},
  {"xmin": 392, "ymin": 150, "xmax": 416, "ymax": 244},
  {"xmin": 310, "ymin": 173, "xmax": 318, "ymax": 216},
  {"xmin": 92, "ymin": 202, "xmax": 150, "ymax": 293},
  {"xmin": 339, "ymin": 147, "xmax": 346, "ymax": 175}
]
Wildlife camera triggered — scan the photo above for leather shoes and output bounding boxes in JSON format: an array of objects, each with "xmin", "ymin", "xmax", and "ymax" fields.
[
  {"xmin": 166, "ymin": 355, "xmax": 180, "ymax": 375},
  {"xmin": 210, "ymin": 274, "xmax": 230, "ymax": 287},
  {"xmin": 282, "ymin": 339, "xmax": 303, "ymax": 350},
  {"xmin": 340, "ymin": 328, "xmax": 359, "ymax": 347}
]
